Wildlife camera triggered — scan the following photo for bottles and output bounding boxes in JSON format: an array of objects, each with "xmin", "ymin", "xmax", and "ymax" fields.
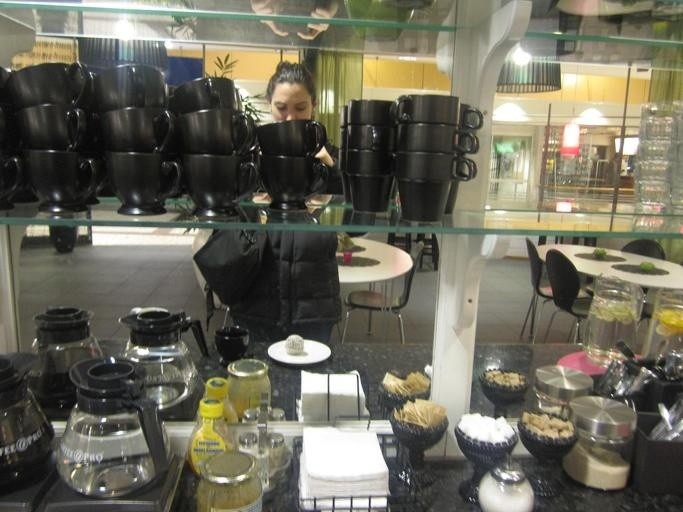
[
  {"xmin": 480, "ymin": 463, "xmax": 535, "ymax": 512},
  {"xmin": 562, "ymin": 393, "xmax": 637, "ymax": 493},
  {"xmin": 185, "ymin": 401, "xmax": 292, "ymax": 511},
  {"xmin": 535, "ymin": 366, "xmax": 593, "ymax": 413},
  {"xmin": 199, "ymin": 358, "xmax": 288, "ymax": 422}
]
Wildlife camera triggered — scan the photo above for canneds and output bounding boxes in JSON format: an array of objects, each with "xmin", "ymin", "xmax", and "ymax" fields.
[
  {"xmin": 197, "ymin": 449, "xmax": 263, "ymax": 512},
  {"xmin": 227, "ymin": 359, "xmax": 271, "ymax": 419}
]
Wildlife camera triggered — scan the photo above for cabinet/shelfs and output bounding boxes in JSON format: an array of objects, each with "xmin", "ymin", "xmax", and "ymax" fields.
[{"xmin": 0, "ymin": 1, "xmax": 681, "ymax": 241}]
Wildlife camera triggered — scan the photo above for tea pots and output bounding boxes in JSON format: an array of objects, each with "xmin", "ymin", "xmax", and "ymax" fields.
[
  {"xmin": 23, "ymin": 307, "xmax": 208, "ymax": 420},
  {"xmin": 0, "ymin": 358, "xmax": 53, "ymax": 494},
  {"xmin": 55, "ymin": 357, "xmax": 175, "ymax": 497}
]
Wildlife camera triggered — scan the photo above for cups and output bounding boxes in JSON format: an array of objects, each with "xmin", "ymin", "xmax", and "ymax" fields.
[
  {"xmin": 48, "ymin": 227, "xmax": 78, "ymax": 253},
  {"xmin": 214, "ymin": 327, "xmax": 249, "ymax": 365},
  {"xmin": 339, "ymin": 93, "xmax": 484, "ymax": 222},
  {"xmin": 642, "ymin": 289, "xmax": 683, "ymax": 371},
  {"xmin": 583, "ymin": 275, "xmax": 645, "ymax": 368},
  {"xmin": 634, "ymin": 100, "xmax": 681, "ymax": 214},
  {"xmin": 0, "ymin": 61, "xmax": 326, "ymax": 216},
  {"xmin": 341, "ymin": 251, "xmax": 355, "ymax": 266}
]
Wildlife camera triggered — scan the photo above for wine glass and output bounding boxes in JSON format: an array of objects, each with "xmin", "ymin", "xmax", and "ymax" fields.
[
  {"xmin": 378, "ymin": 372, "xmax": 431, "ymax": 408},
  {"xmin": 388, "ymin": 406, "xmax": 447, "ymax": 488},
  {"xmin": 454, "ymin": 418, "xmax": 517, "ymax": 503},
  {"xmin": 478, "ymin": 368, "xmax": 529, "ymax": 419},
  {"xmin": 518, "ymin": 413, "xmax": 577, "ymax": 498}
]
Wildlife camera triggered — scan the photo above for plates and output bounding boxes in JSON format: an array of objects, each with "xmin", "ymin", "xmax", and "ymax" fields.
[{"xmin": 269, "ymin": 337, "xmax": 334, "ymax": 364}]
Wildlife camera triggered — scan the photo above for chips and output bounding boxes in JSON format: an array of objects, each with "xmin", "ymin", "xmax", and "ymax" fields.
[
  {"xmin": 383, "ymin": 371, "xmax": 430, "ymax": 396},
  {"xmin": 393, "ymin": 399, "xmax": 447, "ymax": 427}
]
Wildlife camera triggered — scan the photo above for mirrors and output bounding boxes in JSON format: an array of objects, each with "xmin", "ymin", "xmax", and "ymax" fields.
[{"xmin": 0, "ymin": 1, "xmax": 681, "ymax": 422}]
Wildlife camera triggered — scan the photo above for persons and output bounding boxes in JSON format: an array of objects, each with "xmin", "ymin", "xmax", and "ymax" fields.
[{"xmin": 194, "ymin": 61, "xmax": 341, "ymax": 342}]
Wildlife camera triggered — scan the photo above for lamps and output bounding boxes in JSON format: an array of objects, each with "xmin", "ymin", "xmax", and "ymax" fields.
[
  {"xmin": 494, "ymin": 60, "xmax": 562, "ymax": 96},
  {"xmin": 490, "ymin": 76, "xmax": 607, "ymax": 126},
  {"xmin": 76, "ymin": 38, "xmax": 168, "ymax": 75}
]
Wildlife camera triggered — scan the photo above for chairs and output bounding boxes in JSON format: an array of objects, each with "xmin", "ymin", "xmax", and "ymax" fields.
[
  {"xmin": 340, "ymin": 241, "xmax": 425, "ymax": 344},
  {"xmin": 518, "ymin": 235, "xmax": 683, "ymax": 344}
]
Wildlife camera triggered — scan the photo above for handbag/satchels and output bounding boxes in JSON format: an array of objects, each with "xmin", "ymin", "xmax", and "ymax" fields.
[{"xmin": 192, "ymin": 229, "xmax": 276, "ymax": 307}]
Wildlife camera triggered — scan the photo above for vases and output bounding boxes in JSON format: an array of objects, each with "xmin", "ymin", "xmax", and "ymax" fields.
[{"xmin": 48, "ymin": 213, "xmax": 78, "ymax": 254}]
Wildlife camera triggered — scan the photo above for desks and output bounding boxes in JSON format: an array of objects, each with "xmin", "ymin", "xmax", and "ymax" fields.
[{"xmin": 190, "ymin": 228, "xmax": 414, "ymax": 344}]
